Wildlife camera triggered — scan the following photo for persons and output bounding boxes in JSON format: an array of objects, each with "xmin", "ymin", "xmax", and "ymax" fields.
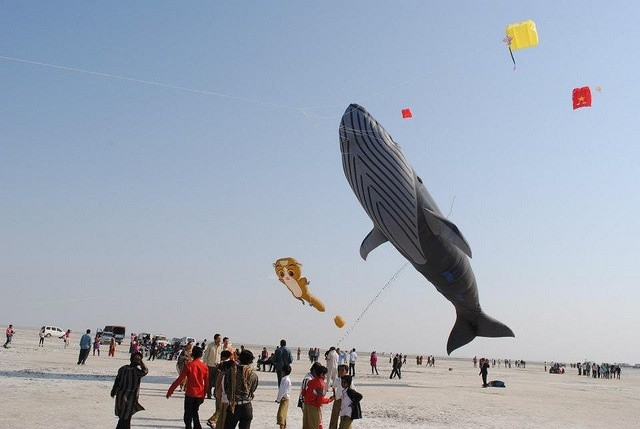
[
  {"xmin": 394, "ymin": 355, "xmax": 403, "ymax": 378},
  {"xmin": 545, "ymin": 360, "xmax": 621, "ymax": 378},
  {"xmin": 129, "ymin": 333, "xmax": 184, "ymax": 361},
  {"xmin": 256, "ymin": 345, "xmax": 281, "ymax": 374},
  {"xmin": 337, "ymin": 375, "xmax": 363, "ymax": 429},
  {"xmin": 390, "ymin": 353, "xmax": 401, "ymax": 379},
  {"xmin": 185, "ymin": 337, "xmax": 208, "ymax": 356},
  {"xmin": 337, "ymin": 348, "xmax": 348, "ymax": 363},
  {"xmin": 109, "ymin": 334, "xmax": 116, "ymax": 357},
  {"xmin": 326, "ymin": 347, "xmax": 339, "ymax": 392},
  {"xmin": 309, "ymin": 347, "xmax": 330, "ymax": 364},
  {"xmin": 111, "ymin": 352, "xmax": 149, "ymax": 429},
  {"xmin": 216, "ymin": 360, "xmax": 240, "ymax": 429},
  {"xmin": 328, "ymin": 364, "xmax": 356, "ymax": 429},
  {"xmin": 223, "ymin": 337, "xmax": 235, "ymax": 359},
  {"xmin": 39, "ymin": 326, "xmax": 46, "ymax": 347},
  {"xmin": 272, "ymin": 340, "xmax": 293, "ymax": 387},
  {"xmin": 473, "ymin": 356, "xmax": 526, "ymax": 368},
  {"xmin": 77, "ymin": 329, "xmax": 91, "ymax": 365},
  {"xmin": 297, "ymin": 347, "xmax": 300, "ymax": 360},
  {"xmin": 207, "ymin": 351, "xmax": 232, "ymax": 428},
  {"xmin": 64, "ymin": 330, "xmax": 71, "ymax": 348},
  {"xmin": 274, "ymin": 365, "xmax": 292, "ymax": 429},
  {"xmin": 301, "ymin": 366, "xmax": 335, "ymax": 429},
  {"xmin": 203, "ymin": 334, "xmax": 224, "ymax": 399},
  {"xmin": 349, "ymin": 348, "xmax": 357, "ymax": 376},
  {"xmin": 166, "ymin": 346, "xmax": 209, "ymax": 429},
  {"xmin": 370, "ymin": 351, "xmax": 381, "ymax": 375},
  {"xmin": 175, "ymin": 342, "xmax": 194, "ymax": 392},
  {"xmin": 301, "ymin": 362, "xmax": 323, "ymax": 429},
  {"xmin": 93, "ymin": 334, "xmax": 101, "ymax": 356},
  {"xmin": 396, "ymin": 353, "xmax": 434, "ymax": 367},
  {"xmin": 479, "ymin": 358, "xmax": 489, "ymax": 387},
  {"xmin": 3, "ymin": 325, "xmax": 15, "ymax": 348},
  {"xmin": 389, "ymin": 351, "xmax": 392, "ymax": 363},
  {"xmin": 224, "ymin": 350, "xmax": 259, "ymax": 429}
]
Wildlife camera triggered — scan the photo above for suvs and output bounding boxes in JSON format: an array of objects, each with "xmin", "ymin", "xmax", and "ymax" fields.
[
  {"xmin": 100, "ymin": 332, "xmax": 113, "ymax": 345},
  {"xmin": 179, "ymin": 337, "xmax": 196, "ymax": 349},
  {"xmin": 152, "ymin": 335, "xmax": 168, "ymax": 347}
]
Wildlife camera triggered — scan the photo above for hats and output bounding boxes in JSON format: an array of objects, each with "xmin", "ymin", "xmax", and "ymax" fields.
[{"xmin": 239, "ymin": 350, "xmax": 255, "ymax": 364}]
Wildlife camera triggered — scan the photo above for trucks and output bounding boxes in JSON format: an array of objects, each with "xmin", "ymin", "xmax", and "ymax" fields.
[{"xmin": 104, "ymin": 326, "xmax": 125, "ymax": 345}]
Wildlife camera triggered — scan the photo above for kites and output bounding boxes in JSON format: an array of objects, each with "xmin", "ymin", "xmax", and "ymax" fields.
[
  {"xmin": 503, "ymin": 19, "xmax": 538, "ymax": 51},
  {"xmin": 572, "ymin": 87, "xmax": 592, "ymax": 110},
  {"xmin": 272, "ymin": 256, "xmax": 325, "ymax": 313},
  {"xmin": 339, "ymin": 102, "xmax": 514, "ymax": 356}
]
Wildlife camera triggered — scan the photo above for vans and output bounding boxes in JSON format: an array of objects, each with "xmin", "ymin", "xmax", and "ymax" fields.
[{"xmin": 42, "ymin": 326, "xmax": 66, "ymax": 338}]
